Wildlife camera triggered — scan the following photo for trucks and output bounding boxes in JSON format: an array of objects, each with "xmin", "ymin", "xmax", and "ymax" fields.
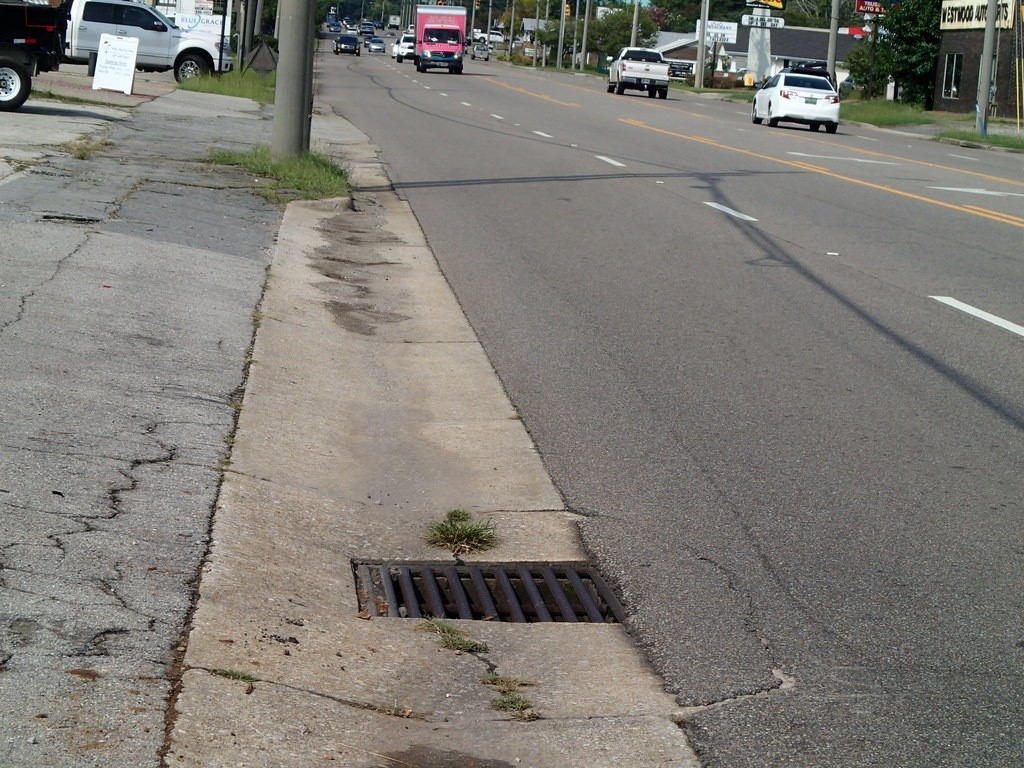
[
  {"xmin": 413, "ymin": 4, "xmax": 467, "ymax": 75},
  {"xmin": 387, "ymin": 15, "xmax": 401, "ymax": 30}
]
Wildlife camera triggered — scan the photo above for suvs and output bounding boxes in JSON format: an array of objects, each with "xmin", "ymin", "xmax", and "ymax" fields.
[
  {"xmin": 778, "ymin": 64, "xmax": 834, "ymax": 87},
  {"xmin": 606, "ymin": 47, "xmax": 672, "ymax": 100}
]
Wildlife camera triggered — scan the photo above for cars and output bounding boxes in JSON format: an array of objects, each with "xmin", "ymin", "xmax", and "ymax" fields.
[
  {"xmin": 327, "ymin": 17, "xmax": 358, "ymax": 33},
  {"xmin": 407, "ymin": 24, "xmax": 414, "ymax": 34},
  {"xmin": 384, "ymin": 32, "xmax": 396, "ymax": 36},
  {"xmin": 397, "ymin": 34, "xmax": 415, "ymax": 63},
  {"xmin": 375, "ymin": 22, "xmax": 385, "ymax": 31},
  {"xmin": 368, "ymin": 38, "xmax": 385, "ymax": 53},
  {"xmin": 751, "ymin": 73, "xmax": 840, "ymax": 133},
  {"xmin": 471, "ymin": 44, "xmax": 489, "ymax": 61},
  {"xmin": 356, "ymin": 22, "xmax": 374, "ymax": 35},
  {"xmin": 332, "ymin": 36, "xmax": 363, "ymax": 56},
  {"xmin": 390, "ymin": 39, "xmax": 400, "ymax": 59},
  {"xmin": 364, "ymin": 35, "xmax": 377, "ymax": 48}
]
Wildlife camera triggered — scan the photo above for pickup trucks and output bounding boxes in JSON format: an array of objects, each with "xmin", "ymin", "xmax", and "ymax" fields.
[
  {"xmin": 0, "ymin": 0, "xmax": 73, "ymax": 112},
  {"xmin": 65, "ymin": 0, "xmax": 235, "ymax": 85},
  {"xmin": 474, "ymin": 30, "xmax": 509, "ymax": 44}
]
